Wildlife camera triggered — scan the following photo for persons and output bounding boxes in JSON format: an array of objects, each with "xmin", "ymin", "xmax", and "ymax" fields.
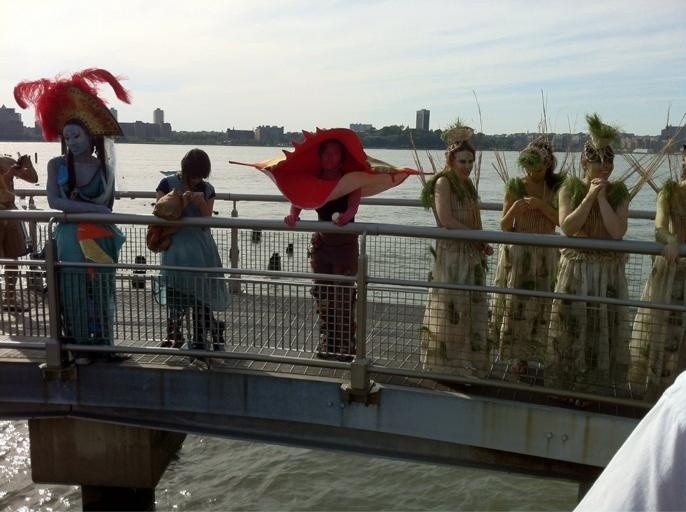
[
  {"xmin": 45, "ymin": 121, "xmax": 125, "ymax": 365},
  {"xmin": 551, "ymin": 137, "xmax": 634, "ymax": 383},
  {"xmin": 574, "ymin": 369, "xmax": 686, "ymax": 512},
  {"xmin": 495, "ymin": 144, "xmax": 564, "ymax": 375},
  {"xmin": 419, "ymin": 142, "xmax": 494, "ymax": 383},
  {"xmin": 626, "ymin": 141, "xmax": 686, "ymax": 389},
  {"xmin": 1, "ymin": 155, "xmax": 39, "ymax": 309},
  {"xmin": 152, "ymin": 148, "xmax": 231, "ymax": 358},
  {"xmin": 280, "ymin": 139, "xmax": 363, "ymax": 368}
]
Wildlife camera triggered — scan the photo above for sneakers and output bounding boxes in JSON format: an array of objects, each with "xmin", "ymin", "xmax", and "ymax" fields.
[
  {"xmin": 161, "ymin": 332, "xmax": 185, "ymax": 348},
  {"xmin": 213, "ymin": 321, "xmax": 225, "ymax": 351}
]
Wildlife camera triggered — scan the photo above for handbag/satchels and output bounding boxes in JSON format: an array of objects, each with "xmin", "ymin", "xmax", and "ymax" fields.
[{"xmin": 146, "ymin": 188, "xmax": 183, "ymax": 252}]
